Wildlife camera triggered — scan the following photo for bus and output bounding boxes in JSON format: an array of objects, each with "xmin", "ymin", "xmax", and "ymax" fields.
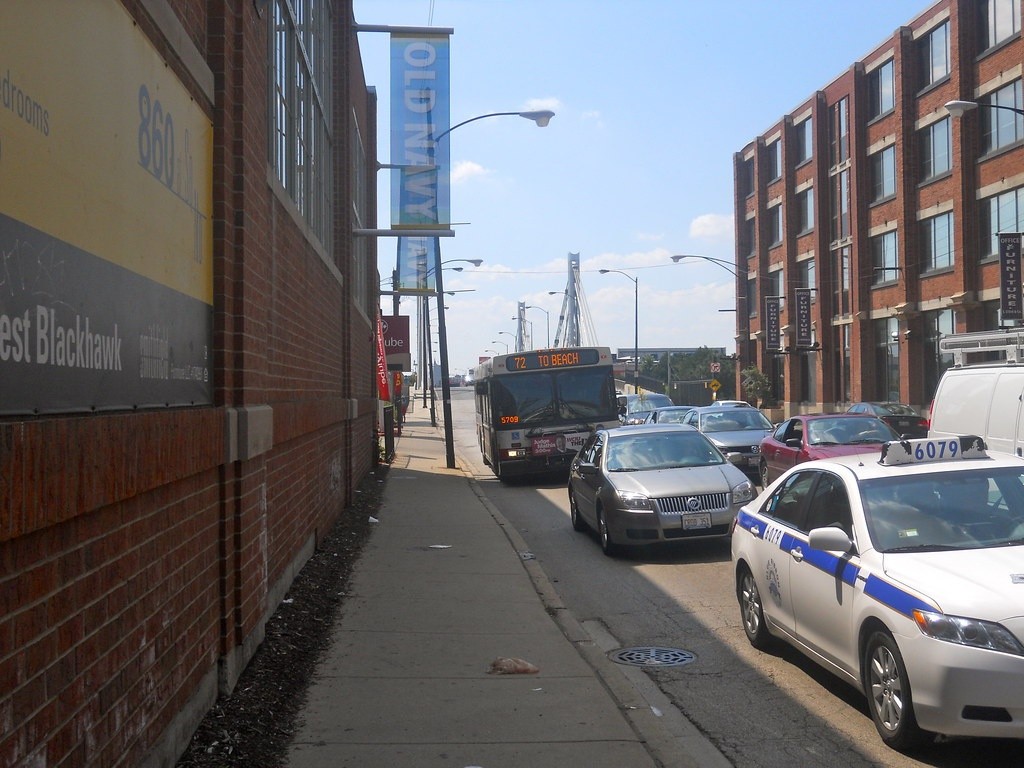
[{"xmin": 473, "ymin": 346, "xmax": 627, "ymax": 484}]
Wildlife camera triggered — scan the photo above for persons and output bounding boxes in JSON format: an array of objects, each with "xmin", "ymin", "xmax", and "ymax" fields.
[{"xmin": 547, "ymin": 434, "xmax": 579, "ymax": 469}]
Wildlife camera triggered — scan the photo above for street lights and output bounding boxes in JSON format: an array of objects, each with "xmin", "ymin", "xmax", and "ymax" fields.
[
  {"xmin": 492, "ymin": 341, "xmax": 508, "ymax": 354},
  {"xmin": 548, "ymin": 291, "xmax": 579, "ymax": 347},
  {"xmin": 417, "ymin": 87, "xmax": 557, "ymax": 468},
  {"xmin": 525, "ymin": 305, "xmax": 549, "ymax": 349},
  {"xmin": 672, "ymin": 254, "xmax": 750, "ymax": 405},
  {"xmin": 512, "ymin": 317, "xmax": 533, "ymax": 351},
  {"xmin": 499, "ymin": 332, "xmax": 517, "ymax": 352},
  {"xmin": 599, "ymin": 269, "xmax": 637, "ymax": 394}
]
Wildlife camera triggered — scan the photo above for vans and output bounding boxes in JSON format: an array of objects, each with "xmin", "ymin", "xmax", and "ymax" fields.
[{"xmin": 927, "ymin": 318, "xmax": 1024, "ymax": 506}]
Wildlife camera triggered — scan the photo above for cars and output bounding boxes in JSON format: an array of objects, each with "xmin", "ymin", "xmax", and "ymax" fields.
[
  {"xmin": 730, "ymin": 435, "xmax": 1024, "ymax": 755},
  {"xmin": 839, "ymin": 401, "xmax": 927, "ymax": 440},
  {"xmin": 756, "ymin": 412, "xmax": 915, "ymax": 493},
  {"xmin": 567, "ymin": 424, "xmax": 758, "ymax": 558},
  {"xmin": 679, "ymin": 405, "xmax": 783, "ymax": 475},
  {"xmin": 643, "ymin": 405, "xmax": 700, "ymax": 425},
  {"xmin": 614, "ymin": 393, "xmax": 676, "ymax": 426},
  {"xmin": 709, "ymin": 400, "xmax": 751, "ymax": 407}
]
